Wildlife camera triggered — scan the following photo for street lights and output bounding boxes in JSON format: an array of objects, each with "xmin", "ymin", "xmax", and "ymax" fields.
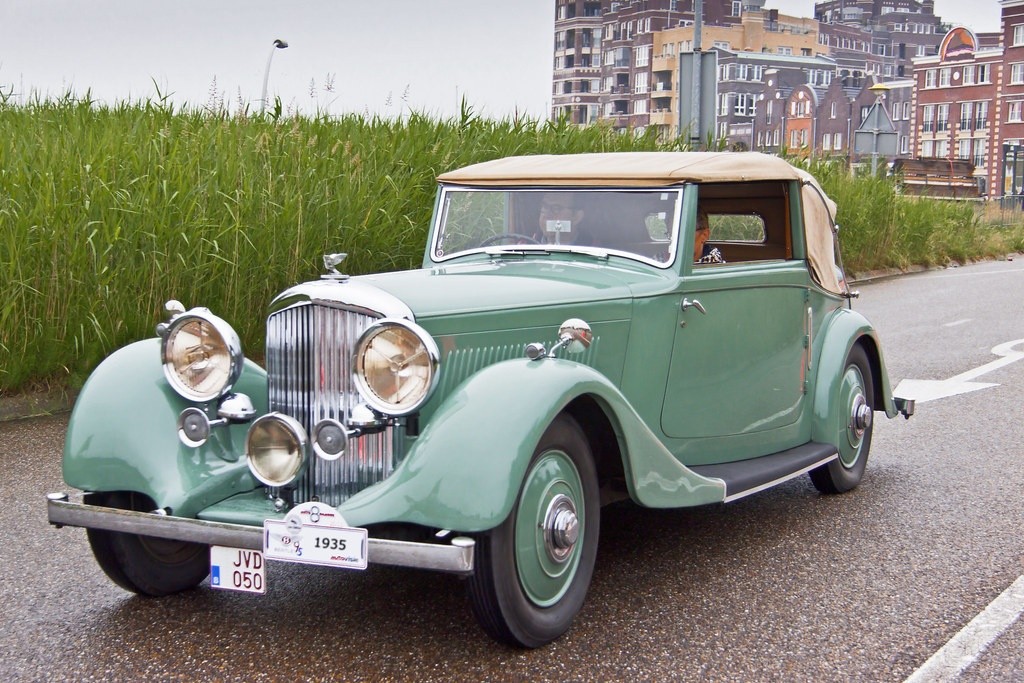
[{"xmin": 254, "ymin": 39, "xmax": 289, "ymax": 123}]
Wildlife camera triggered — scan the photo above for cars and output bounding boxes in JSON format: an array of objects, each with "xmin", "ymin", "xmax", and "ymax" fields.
[{"xmin": 46, "ymin": 150, "xmax": 917, "ymax": 651}]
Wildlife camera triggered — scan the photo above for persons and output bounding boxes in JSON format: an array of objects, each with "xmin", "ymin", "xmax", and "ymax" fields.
[
  {"xmin": 649, "ymin": 201, "xmax": 722, "ymax": 264},
  {"xmin": 515, "ymin": 192, "xmax": 600, "ymax": 247}
]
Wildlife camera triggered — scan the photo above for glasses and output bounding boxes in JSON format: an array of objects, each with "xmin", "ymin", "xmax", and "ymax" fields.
[
  {"xmin": 537, "ymin": 202, "xmax": 575, "ymax": 214},
  {"xmin": 665, "ymin": 228, "xmax": 703, "ymax": 240}
]
